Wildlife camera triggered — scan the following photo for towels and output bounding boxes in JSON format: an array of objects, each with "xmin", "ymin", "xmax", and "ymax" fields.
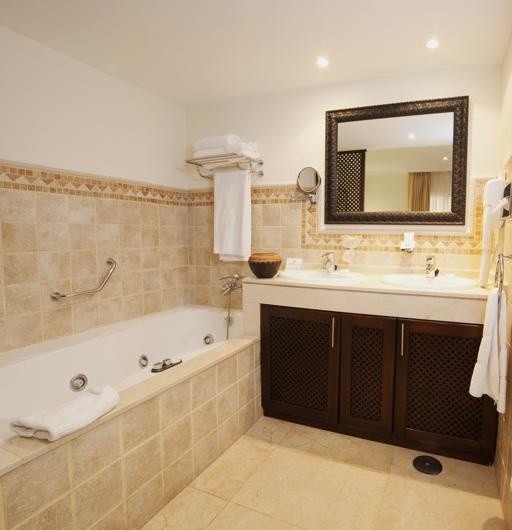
[
  {"xmin": 8, "ymin": 382, "xmax": 120, "ymax": 443},
  {"xmin": 193, "ymin": 134, "xmax": 261, "ymax": 263},
  {"xmin": 467, "ymin": 179, "xmax": 510, "ymax": 421}
]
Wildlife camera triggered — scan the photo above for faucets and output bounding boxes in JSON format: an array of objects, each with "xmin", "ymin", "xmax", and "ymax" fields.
[
  {"xmin": 321, "ymin": 253, "xmax": 336, "ymax": 272},
  {"xmin": 424, "ymin": 256, "xmax": 435, "ymax": 274},
  {"xmin": 222, "ymin": 286, "xmax": 236, "ymax": 296}
]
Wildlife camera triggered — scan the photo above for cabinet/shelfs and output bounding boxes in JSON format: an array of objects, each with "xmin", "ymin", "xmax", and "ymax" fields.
[{"xmin": 260, "ymin": 304, "xmax": 499, "ymax": 470}]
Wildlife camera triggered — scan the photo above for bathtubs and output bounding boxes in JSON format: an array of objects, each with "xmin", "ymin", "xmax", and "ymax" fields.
[{"xmin": 0, "ymin": 306, "xmax": 245, "ymax": 444}]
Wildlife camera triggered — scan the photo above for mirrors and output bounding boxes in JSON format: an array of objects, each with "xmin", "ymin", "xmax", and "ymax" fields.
[{"xmin": 324, "ymin": 95, "xmax": 468, "ymax": 227}]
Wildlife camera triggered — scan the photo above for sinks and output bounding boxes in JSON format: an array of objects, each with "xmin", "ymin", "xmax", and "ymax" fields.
[
  {"xmin": 278, "ymin": 271, "xmax": 361, "ymax": 284},
  {"xmin": 382, "ymin": 274, "xmax": 476, "ymax": 290}
]
